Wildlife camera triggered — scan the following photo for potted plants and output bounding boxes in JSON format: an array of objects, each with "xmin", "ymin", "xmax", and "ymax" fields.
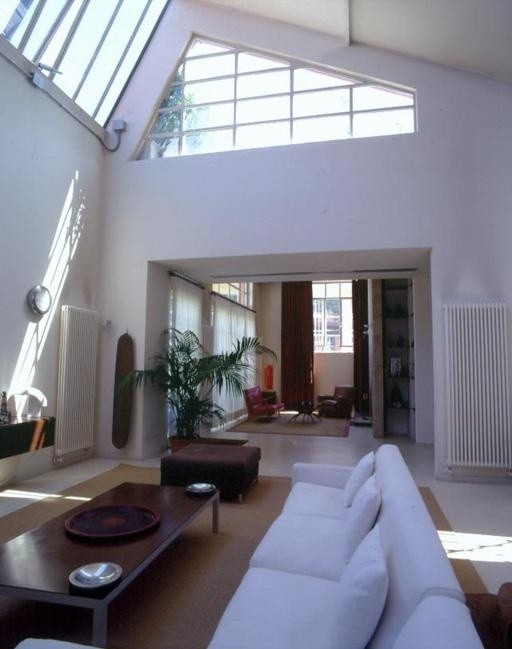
[{"xmin": 111, "ymin": 327, "xmax": 278, "ymax": 453}]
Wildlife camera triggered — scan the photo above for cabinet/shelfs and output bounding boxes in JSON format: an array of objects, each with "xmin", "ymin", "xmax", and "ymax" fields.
[{"xmin": 382, "ymin": 279, "xmax": 415, "ymax": 410}]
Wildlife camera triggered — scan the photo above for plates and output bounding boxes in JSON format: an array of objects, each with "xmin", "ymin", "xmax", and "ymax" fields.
[
  {"xmin": 68, "ymin": 560, "xmax": 123, "ymax": 590},
  {"xmin": 185, "ymin": 482, "xmax": 217, "ymax": 494}
]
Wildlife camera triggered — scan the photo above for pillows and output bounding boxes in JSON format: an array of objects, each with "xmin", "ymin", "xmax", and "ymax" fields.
[{"xmin": 331, "ymin": 450, "xmax": 390, "ymax": 649}]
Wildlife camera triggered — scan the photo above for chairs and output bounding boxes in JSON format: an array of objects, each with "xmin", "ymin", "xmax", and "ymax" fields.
[
  {"xmin": 241, "ymin": 385, "xmax": 284, "ymax": 424},
  {"xmin": 316, "ymin": 385, "xmax": 354, "ymax": 418}
]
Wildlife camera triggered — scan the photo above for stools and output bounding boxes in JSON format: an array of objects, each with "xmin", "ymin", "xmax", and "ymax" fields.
[{"xmin": 160, "ymin": 443, "xmax": 262, "ymax": 505}]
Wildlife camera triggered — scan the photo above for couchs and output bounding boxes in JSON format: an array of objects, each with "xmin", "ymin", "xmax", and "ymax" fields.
[{"xmin": 206, "ymin": 443, "xmax": 484, "ymax": 649}]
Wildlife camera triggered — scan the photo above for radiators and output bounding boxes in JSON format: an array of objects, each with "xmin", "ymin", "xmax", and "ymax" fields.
[
  {"xmin": 443, "ymin": 302, "xmax": 512, "ymax": 477},
  {"xmin": 53, "ymin": 303, "xmax": 100, "ymax": 464}
]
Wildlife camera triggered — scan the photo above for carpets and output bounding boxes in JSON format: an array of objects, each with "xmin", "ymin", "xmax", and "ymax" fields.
[
  {"xmin": 225, "ymin": 414, "xmax": 352, "ymax": 437},
  {"xmin": 0, "ymin": 462, "xmax": 511, "ymax": 648}
]
